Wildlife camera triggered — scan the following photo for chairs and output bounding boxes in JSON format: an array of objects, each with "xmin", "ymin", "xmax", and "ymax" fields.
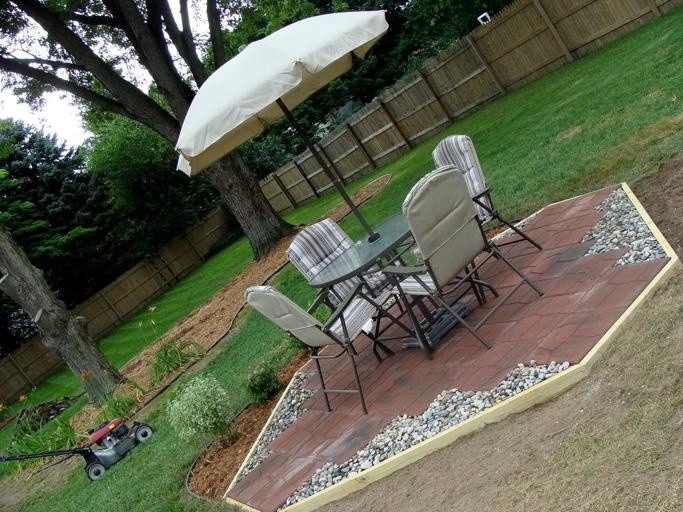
[
  {"xmin": 243, "ymin": 284, "xmax": 415, "ymax": 413},
  {"xmin": 285, "ymin": 216, "xmax": 439, "ymax": 361},
  {"xmin": 430, "ymin": 135, "xmax": 543, "ymax": 302},
  {"xmin": 383, "ymin": 162, "xmax": 543, "ymax": 350}
]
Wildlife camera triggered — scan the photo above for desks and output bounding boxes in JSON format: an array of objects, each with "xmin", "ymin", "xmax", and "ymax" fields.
[{"xmin": 309, "ymin": 214, "xmax": 500, "ymax": 361}]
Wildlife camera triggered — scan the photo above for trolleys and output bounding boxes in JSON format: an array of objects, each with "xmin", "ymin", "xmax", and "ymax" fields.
[{"xmin": 0, "ymin": 417, "xmax": 153, "ymax": 482}]
